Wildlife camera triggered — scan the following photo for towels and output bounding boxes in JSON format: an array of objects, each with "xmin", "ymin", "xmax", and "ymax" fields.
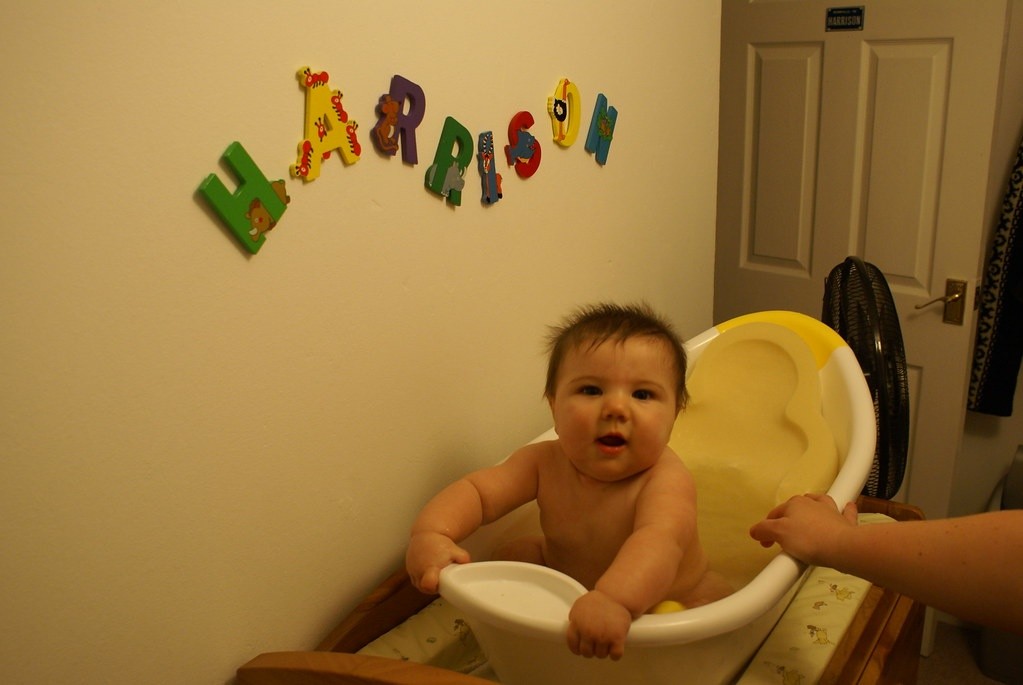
[{"xmin": 966, "ymin": 133, "xmax": 1023, "ymax": 417}]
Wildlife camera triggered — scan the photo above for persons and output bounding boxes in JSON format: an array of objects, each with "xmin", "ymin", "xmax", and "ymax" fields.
[
  {"xmin": 750, "ymin": 494, "xmax": 1023, "ymax": 631},
  {"xmin": 407, "ymin": 301, "xmax": 735, "ymax": 661}
]
